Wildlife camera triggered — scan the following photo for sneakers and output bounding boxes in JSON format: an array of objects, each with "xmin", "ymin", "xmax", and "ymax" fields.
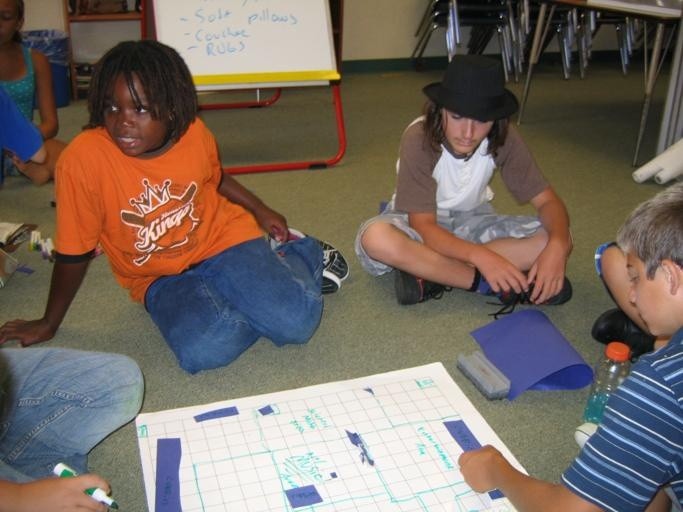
[
  {"xmin": 268, "ymin": 228, "xmax": 350, "ymax": 281},
  {"xmin": 395, "ymin": 269, "xmax": 452, "ymax": 305},
  {"xmin": 486, "ymin": 271, "xmax": 572, "ymax": 320},
  {"xmin": 322, "ymin": 270, "xmax": 341, "ymax": 294},
  {"xmin": 591, "ymin": 308, "xmax": 657, "ymax": 363}
]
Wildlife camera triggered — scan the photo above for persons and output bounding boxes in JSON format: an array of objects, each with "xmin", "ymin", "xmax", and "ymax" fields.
[
  {"xmin": 0, "ymin": 38, "xmax": 350, "ymax": 375},
  {"xmin": 0, "ymin": 85, "xmax": 47, "ymax": 164},
  {"xmin": 592, "ymin": 240, "xmax": 671, "ymax": 364},
  {"xmin": 0, "ymin": 346, "xmax": 146, "ymax": 512},
  {"xmin": 0, "ymin": 1, "xmax": 67, "ymax": 185},
  {"xmin": 353, "ymin": 54, "xmax": 573, "ymax": 320},
  {"xmin": 458, "ymin": 182, "xmax": 683, "ymax": 512}
]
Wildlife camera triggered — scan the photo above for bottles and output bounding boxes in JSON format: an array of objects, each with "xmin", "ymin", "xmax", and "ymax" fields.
[{"xmin": 581, "ymin": 342, "xmax": 634, "ymax": 424}]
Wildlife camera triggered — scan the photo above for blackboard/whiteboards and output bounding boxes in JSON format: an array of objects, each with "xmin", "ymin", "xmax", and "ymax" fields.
[{"xmin": 150, "ymin": 1, "xmax": 341, "ymax": 93}]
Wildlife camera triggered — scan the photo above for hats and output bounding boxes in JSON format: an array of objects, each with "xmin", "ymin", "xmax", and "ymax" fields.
[{"xmin": 422, "ymin": 54, "xmax": 519, "ymax": 122}]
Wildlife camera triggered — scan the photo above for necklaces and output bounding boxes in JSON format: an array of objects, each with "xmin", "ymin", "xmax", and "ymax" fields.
[{"xmin": 142, "ymin": 137, "xmax": 168, "ymax": 155}]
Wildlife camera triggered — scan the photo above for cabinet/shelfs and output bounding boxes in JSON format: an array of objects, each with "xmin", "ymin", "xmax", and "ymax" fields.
[{"xmin": 61, "ymin": 0, "xmax": 344, "ymax": 100}]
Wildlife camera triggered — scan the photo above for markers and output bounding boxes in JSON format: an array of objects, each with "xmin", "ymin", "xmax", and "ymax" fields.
[
  {"xmin": 52, "ymin": 461, "xmax": 119, "ymax": 511},
  {"xmin": 29, "ymin": 230, "xmax": 56, "ymax": 263}
]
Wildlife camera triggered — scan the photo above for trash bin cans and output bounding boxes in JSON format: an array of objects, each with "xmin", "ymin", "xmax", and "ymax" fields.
[{"xmin": 19, "ymin": 28, "xmax": 71, "ymax": 107}]
